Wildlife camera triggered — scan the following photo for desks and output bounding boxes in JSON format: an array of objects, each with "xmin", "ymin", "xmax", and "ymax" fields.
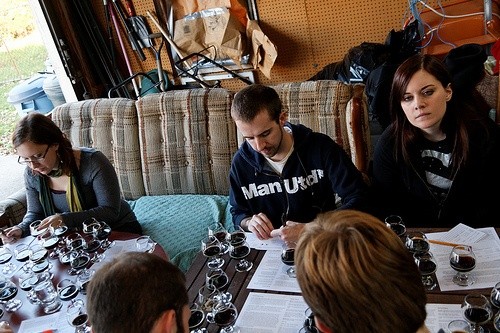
[
  {"xmin": 0, "ymin": 230, "xmax": 171, "ymax": 333},
  {"xmin": 183, "ymin": 227, "xmax": 500, "ymax": 333}
]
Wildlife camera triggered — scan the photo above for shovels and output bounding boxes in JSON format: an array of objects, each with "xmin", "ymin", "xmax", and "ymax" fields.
[
  {"xmin": 124, "ymin": 0, "xmax": 153, "ymax": 50},
  {"xmin": 148, "ymin": 33, "xmax": 164, "ymax": 91}
]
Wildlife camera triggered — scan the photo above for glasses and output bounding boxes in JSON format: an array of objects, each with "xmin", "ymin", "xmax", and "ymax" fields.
[{"xmin": 18, "ymin": 144, "xmax": 51, "ymax": 165}]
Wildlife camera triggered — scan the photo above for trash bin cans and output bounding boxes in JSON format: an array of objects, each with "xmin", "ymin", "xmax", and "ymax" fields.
[
  {"xmin": 7, "ymin": 74, "xmax": 55, "ymax": 120},
  {"xmin": 43, "ymin": 59, "xmax": 55, "ymax": 73},
  {"xmin": 42, "ymin": 72, "xmax": 66, "ymax": 108}
]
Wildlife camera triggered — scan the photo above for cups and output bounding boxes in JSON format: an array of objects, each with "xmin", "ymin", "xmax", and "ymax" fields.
[
  {"xmin": 404, "ymin": 231, "xmax": 431, "ymax": 254},
  {"xmin": 225, "ymin": 224, "xmax": 247, "ymax": 247},
  {"xmin": 135, "ymin": 236, "xmax": 156, "ymax": 254},
  {"xmin": 297, "ymin": 306, "xmax": 320, "ymax": 333},
  {"xmin": 384, "ymin": 215, "xmax": 407, "ymax": 238},
  {"xmin": 28, "ymin": 271, "xmax": 63, "ymax": 314}
]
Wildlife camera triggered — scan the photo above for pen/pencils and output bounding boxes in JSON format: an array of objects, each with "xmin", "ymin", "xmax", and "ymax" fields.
[
  {"xmin": 429, "ymin": 240, "xmax": 472, "ymax": 251},
  {"xmin": 37, "ymin": 329, "xmax": 57, "ymax": 333},
  {"xmin": 0, "ymin": 228, "xmax": 18, "ymax": 241},
  {"xmin": 281, "ymin": 213, "xmax": 286, "ymax": 227}
]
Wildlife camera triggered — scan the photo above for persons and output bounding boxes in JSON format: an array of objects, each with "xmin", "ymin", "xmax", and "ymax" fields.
[
  {"xmin": 374, "ymin": 54, "xmax": 500, "ymax": 229},
  {"xmin": 229, "ymin": 85, "xmax": 376, "ymax": 241},
  {"xmin": 86, "ymin": 252, "xmax": 191, "ymax": 333},
  {"xmin": 0, "ymin": 114, "xmax": 143, "ymax": 244},
  {"xmin": 294, "ymin": 210, "xmax": 429, "ymax": 333}
]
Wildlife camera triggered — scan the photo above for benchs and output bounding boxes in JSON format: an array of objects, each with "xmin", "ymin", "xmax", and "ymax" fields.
[{"xmin": 1, "ymin": 80, "xmax": 372, "ymax": 274}]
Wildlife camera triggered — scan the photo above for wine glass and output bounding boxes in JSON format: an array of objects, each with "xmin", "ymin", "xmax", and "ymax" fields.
[
  {"xmin": 448, "ymin": 244, "xmax": 477, "ymax": 287},
  {"xmin": 280, "ymin": 240, "xmax": 297, "ymax": 278},
  {"xmin": 413, "ymin": 249, "xmax": 438, "ymax": 291},
  {"xmin": 212, "ymin": 299, "xmax": 241, "ymax": 333},
  {"xmin": 187, "ymin": 301, "xmax": 209, "ymax": 333},
  {"xmin": 208, "ymin": 221, "xmax": 229, "ymax": 256},
  {"xmin": 446, "ymin": 281, "xmax": 500, "ymax": 333},
  {"xmin": 0, "ymin": 218, "xmax": 116, "ymax": 333},
  {"xmin": 226, "ymin": 237, "xmax": 254, "ymax": 273},
  {"xmin": 199, "ymin": 284, "xmax": 222, "ymax": 324},
  {"xmin": 205, "ymin": 266, "xmax": 233, "ymax": 302},
  {"xmin": 200, "ymin": 235, "xmax": 225, "ymax": 269}
]
